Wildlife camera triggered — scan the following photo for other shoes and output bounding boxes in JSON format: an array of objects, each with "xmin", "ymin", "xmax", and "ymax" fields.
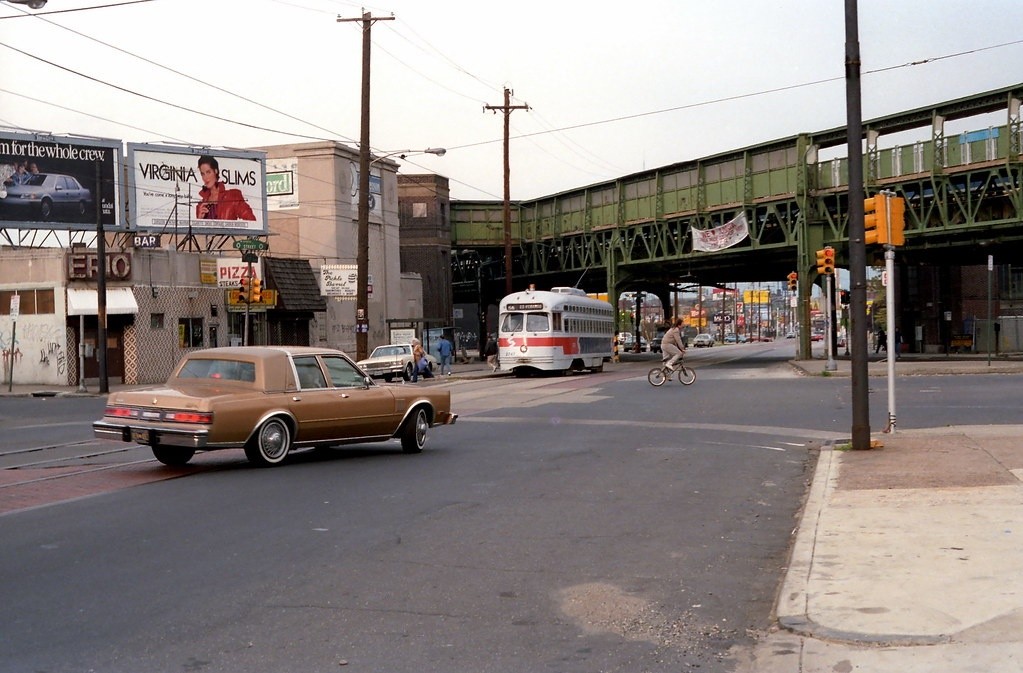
[
  {"xmin": 492, "ymin": 366, "xmax": 497, "ymax": 372},
  {"xmin": 409, "ymin": 380, "xmax": 417, "ymax": 383},
  {"xmin": 448, "ymin": 372, "xmax": 451, "ymax": 376},
  {"xmin": 665, "ymin": 363, "xmax": 674, "ymax": 371}
]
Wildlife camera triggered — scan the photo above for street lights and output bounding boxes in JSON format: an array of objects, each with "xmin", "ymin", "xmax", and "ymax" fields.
[{"xmin": 355, "ymin": 146, "xmax": 448, "ymax": 361}]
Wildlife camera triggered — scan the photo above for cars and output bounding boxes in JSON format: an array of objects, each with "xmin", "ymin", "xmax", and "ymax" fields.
[
  {"xmin": 786, "ymin": 329, "xmax": 845, "ymax": 347},
  {"xmin": 692, "ymin": 333, "xmax": 716, "ymax": 348},
  {"xmin": 723, "ymin": 333, "xmax": 772, "ymax": 344},
  {"xmin": 618, "ymin": 331, "xmax": 689, "ymax": 353},
  {"xmin": 0, "ymin": 171, "xmax": 94, "ymax": 220},
  {"xmin": 91, "ymin": 344, "xmax": 460, "ymax": 467},
  {"xmin": 353, "ymin": 344, "xmax": 438, "ymax": 383}
]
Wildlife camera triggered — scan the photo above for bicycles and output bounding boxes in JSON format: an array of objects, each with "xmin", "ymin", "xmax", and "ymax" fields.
[{"xmin": 647, "ymin": 351, "xmax": 697, "ymax": 387}]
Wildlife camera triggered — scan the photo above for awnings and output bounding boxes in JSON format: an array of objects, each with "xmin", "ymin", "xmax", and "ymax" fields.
[{"xmin": 68, "ymin": 288, "xmax": 139, "ymax": 316}]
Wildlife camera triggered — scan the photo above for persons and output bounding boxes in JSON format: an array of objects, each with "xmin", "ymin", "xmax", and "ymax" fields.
[
  {"xmin": 661, "ymin": 319, "xmax": 686, "ymax": 381},
  {"xmin": 460, "ymin": 335, "xmax": 468, "ymax": 356},
  {"xmin": 437, "ymin": 335, "xmax": 453, "ymax": 375},
  {"xmin": 483, "ymin": 336, "xmax": 499, "ymax": 372},
  {"xmin": 195, "ymin": 156, "xmax": 257, "ymax": 221},
  {"xmin": 4, "ymin": 162, "xmax": 38, "ymax": 186},
  {"xmin": 875, "ymin": 327, "xmax": 887, "ymax": 354},
  {"xmin": 409, "ymin": 339, "xmax": 433, "ymax": 382},
  {"xmin": 895, "ymin": 327, "xmax": 901, "ymax": 358}
]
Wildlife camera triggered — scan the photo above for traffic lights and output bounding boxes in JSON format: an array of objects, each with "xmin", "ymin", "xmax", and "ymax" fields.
[
  {"xmin": 845, "ymin": 291, "xmax": 851, "ymax": 305},
  {"xmin": 787, "ymin": 273, "xmax": 791, "ymax": 291},
  {"xmin": 790, "ymin": 273, "xmax": 798, "ymax": 291},
  {"xmin": 238, "ymin": 278, "xmax": 250, "ymax": 304},
  {"xmin": 840, "ymin": 290, "xmax": 846, "ymax": 304},
  {"xmin": 815, "ymin": 250, "xmax": 825, "ymax": 275},
  {"xmin": 630, "ymin": 313, "xmax": 634, "ymax": 324},
  {"xmin": 252, "ymin": 277, "xmax": 265, "ymax": 304},
  {"xmin": 824, "ymin": 249, "xmax": 834, "ymax": 275},
  {"xmin": 864, "ymin": 194, "xmax": 888, "ymax": 246}
]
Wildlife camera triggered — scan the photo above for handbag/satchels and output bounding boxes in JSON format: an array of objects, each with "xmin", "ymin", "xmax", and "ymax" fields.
[{"xmin": 418, "ymin": 358, "xmax": 428, "ymax": 372}]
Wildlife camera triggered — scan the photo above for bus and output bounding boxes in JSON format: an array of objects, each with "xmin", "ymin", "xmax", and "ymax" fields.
[{"xmin": 497, "ymin": 283, "xmax": 615, "ymax": 379}]
